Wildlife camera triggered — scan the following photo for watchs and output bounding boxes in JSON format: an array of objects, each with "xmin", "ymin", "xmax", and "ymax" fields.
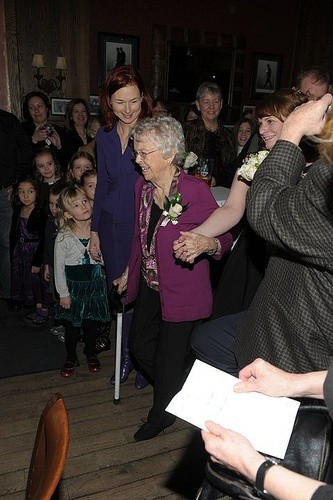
[{"xmin": 254, "ymin": 459, "xmax": 282, "ymax": 500}]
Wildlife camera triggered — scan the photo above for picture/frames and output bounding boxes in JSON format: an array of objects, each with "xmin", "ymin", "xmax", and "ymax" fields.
[
  {"xmin": 242, "ymin": 104, "xmax": 257, "ymax": 120},
  {"xmin": 98, "ymin": 32, "xmax": 139, "ymax": 94},
  {"xmin": 251, "ymin": 53, "xmax": 284, "ymax": 101},
  {"xmin": 89, "ymin": 94, "xmax": 103, "ymax": 116},
  {"xmin": 50, "ymin": 97, "xmax": 73, "ymax": 116}
]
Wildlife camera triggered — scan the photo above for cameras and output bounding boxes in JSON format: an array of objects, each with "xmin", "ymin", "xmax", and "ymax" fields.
[{"xmin": 40, "ymin": 124, "xmax": 54, "ymax": 136}]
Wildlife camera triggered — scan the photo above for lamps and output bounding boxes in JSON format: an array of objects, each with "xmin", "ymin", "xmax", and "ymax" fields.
[{"xmin": 32, "ymin": 54, "xmax": 67, "ymax": 96}]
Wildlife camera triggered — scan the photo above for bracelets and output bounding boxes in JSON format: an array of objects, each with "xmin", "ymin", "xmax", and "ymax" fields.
[
  {"xmin": 208, "ymin": 239, "xmax": 219, "ymax": 256},
  {"xmin": 121, "ymin": 273, "xmax": 128, "ymax": 280}
]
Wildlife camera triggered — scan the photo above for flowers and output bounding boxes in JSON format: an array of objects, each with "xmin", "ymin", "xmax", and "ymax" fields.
[
  {"xmin": 161, "ymin": 191, "xmax": 188, "ymax": 228},
  {"xmin": 179, "ymin": 152, "xmax": 199, "ymax": 172},
  {"xmin": 238, "ymin": 150, "xmax": 270, "ymax": 181}
]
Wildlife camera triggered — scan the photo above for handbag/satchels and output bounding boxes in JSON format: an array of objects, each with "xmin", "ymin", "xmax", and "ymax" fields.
[{"xmin": 205, "ymin": 404, "xmax": 333, "ymax": 500}]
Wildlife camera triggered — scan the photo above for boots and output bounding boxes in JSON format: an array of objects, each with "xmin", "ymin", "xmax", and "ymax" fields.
[
  {"xmin": 109, "ymin": 313, "xmax": 133, "ymax": 385},
  {"xmin": 135, "ymin": 371, "xmax": 150, "ymax": 389}
]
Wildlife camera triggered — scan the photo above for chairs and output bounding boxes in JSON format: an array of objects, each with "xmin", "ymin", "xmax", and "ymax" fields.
[{"xmin": 26, "ymin": 393, "xmax": 68, "ymax": 499}]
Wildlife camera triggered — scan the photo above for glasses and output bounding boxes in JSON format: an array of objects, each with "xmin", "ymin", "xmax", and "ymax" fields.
[{"xmin": 132, "ymin": 150, "xmax": 156, "ymax": 158}]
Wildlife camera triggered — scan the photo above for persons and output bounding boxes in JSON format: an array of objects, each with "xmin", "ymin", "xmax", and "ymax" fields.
[
  {"xmin": 201, "ymin": 357, "xmax": 333, "ymax": 500},
  {"xmin": 0, "ymin": 64, "xmax": 333, "ymax": 440}
]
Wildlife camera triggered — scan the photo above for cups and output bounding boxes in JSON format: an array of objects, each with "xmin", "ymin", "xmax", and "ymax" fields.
[{"xmin": 196, "ymin": 159, "xmax": 215, "ymax": 188}]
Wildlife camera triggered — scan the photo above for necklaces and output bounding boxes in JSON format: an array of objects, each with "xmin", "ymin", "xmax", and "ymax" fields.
[{"xmin": 119, "ymin": 125, "xmax": 129, "ymax": 150}]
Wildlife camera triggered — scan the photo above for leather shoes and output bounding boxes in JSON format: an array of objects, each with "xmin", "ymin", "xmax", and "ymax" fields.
[
  {"xmin": 61, "ymin": 358, "xmax": 79, "ymax": 377},
  {"xmin": 87, "ymin": 356, "xmax": 101, "ymax": 372}
]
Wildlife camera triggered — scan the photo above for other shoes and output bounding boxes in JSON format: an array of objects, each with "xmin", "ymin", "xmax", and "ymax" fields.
[
  {"xmin": 22, "ymin": 310, "xmax": 50, "ymax": 327},
  {"xmin": 132, "ymin": 416, "xmax": 179, "ymax": 440},
  {"xmin": 50, "ymin": 324, "xmax": 65, "ymax": 342}
]
